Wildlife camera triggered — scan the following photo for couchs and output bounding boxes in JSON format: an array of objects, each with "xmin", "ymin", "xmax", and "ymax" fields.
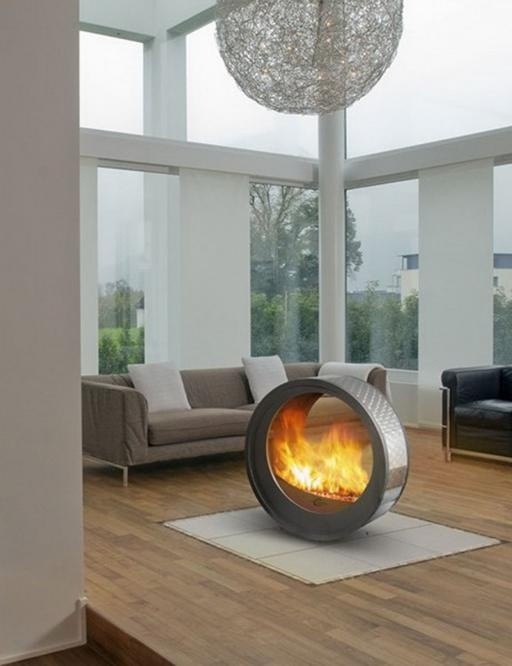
[{"xmin": 80, "ymin": 353, "xmax": 387, "ymax": 489}]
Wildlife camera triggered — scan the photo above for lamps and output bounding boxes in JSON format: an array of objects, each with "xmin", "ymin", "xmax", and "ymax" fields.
[{"xmin": 215, "ymin": 0, "xmax": 403, "ymax": 115}]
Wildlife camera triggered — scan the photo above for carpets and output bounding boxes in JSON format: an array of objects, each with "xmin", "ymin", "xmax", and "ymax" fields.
[{"xmin": 160, "ymin": 504, "xmax": 502, "ymax": 585}]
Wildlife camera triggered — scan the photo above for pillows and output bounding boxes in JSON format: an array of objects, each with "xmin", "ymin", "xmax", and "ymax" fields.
[
  {"xmin": 242, "ymin": 350, "xmax": 289, "ymax": 401},
  {"xmin": 125, "ymin": 359, "xmax": 194, "ymax": 411}
]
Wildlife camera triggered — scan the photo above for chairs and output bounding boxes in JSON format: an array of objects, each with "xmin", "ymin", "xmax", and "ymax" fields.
[{"xmin": 439, "ymin": 363, "xmax": 512, "ymax": 463}]
